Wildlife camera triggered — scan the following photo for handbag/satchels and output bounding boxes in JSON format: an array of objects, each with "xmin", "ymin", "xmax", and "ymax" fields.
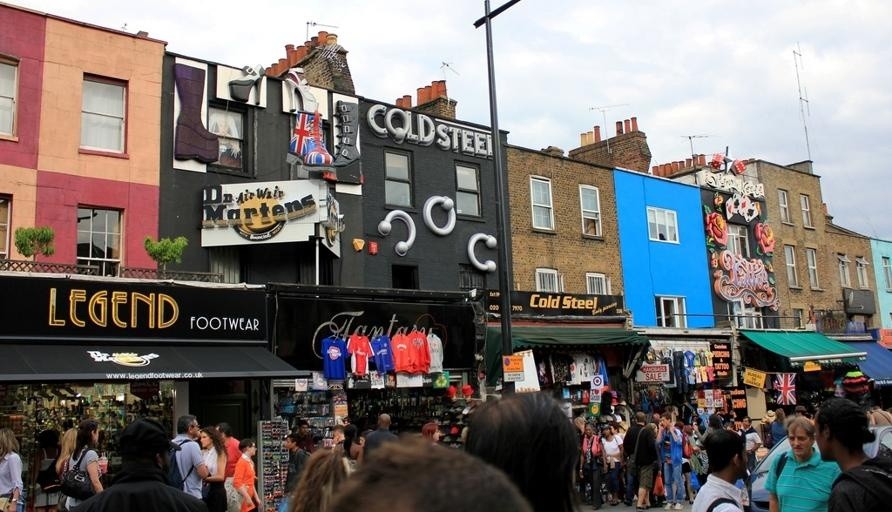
[
  {"xmin": 224, "ymin": 477, "xmax": 250, "ymax": 512},
  {"xmin": 0, "ymin": 496, "xmax": 9, "ymax": 512},
  {"xmin": 59, "ymin": 468, "xmax": 95, "ymax": 501},
  {"xmin": 202, "ymin": 481, "xmax": 211, "ymax": 499},
  {"xmin": 625, "ymin": 455, "xmax": 639, "ymax": 478}
]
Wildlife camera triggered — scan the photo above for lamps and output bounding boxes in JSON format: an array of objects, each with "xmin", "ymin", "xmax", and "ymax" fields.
[{"xmin": 12, "ymin": 384, "xmax": 80, "ymax": 405}]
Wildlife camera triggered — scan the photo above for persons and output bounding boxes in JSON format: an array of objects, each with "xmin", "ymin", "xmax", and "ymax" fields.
[
  {"xmin": 34, "ymin": 383, "xmax": 891, "ymax": 512},
  {"xmin": 1, "ymin": 426, "xmax": 26, "ymax": 512}
]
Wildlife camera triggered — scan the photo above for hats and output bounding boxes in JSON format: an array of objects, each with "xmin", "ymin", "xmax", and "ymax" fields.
[
  {"xmin": 447, "ymin": 385, "xmax": 457, "ymax": 398},
  {"xmin": 118, "ymin": 418, "xmax": 181, "ymax": 461},
  {"xmin": 460, "ymin": 384, "xmax": 476, "ymax": 396},
  {"xmin": 764, "ymin": 409, "xmax": 778, "ymax": 423}
]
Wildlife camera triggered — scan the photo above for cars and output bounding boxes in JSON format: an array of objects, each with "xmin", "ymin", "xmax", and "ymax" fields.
[{"xmin": 741, "ymin": 423, "xmax": 892, "ymax": 512}]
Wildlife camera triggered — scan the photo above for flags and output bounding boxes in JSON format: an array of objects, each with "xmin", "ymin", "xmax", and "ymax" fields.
[{"xmin": 776, "ymin": 373, "xmax": 796, "ymax": 406}]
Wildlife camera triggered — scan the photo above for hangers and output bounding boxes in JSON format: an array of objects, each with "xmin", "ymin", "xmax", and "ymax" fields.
[
  {"xmin": 357, "ymin": 327, "xmax": 434, "ymax": 339},
  {"xmin": 328, "ymin": 331, "xmax": 338, "ymax": 343}
]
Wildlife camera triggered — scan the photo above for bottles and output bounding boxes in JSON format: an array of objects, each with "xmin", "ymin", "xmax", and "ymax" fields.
[
  {"xmin": 100, "ymin": 452, "xmax": 109, "ymax": 475},
  {"xmin": 735, "ymin": 480, "xmax": 750, "ymax": 506}
]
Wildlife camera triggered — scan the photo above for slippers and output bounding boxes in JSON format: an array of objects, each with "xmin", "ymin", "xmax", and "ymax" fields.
[{"xmin": 433, "ymin": 404, "xmax": 478, "ymax": 425}]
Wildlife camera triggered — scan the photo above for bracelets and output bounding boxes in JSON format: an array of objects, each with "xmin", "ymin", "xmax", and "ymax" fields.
[{"xmin": 11, "ymin": 498, "xmax": 18, "ymax": 502}]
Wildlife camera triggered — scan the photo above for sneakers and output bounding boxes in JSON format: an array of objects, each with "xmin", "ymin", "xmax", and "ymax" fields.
[{"xmin": 579, "ymin": 497, "xmax": 685, "ymax": 511}]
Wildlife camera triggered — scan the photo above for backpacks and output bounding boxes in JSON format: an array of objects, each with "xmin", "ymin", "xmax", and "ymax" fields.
[
  {"xmin": 763, "ymin": 433, "xmax": 773, "ymax": 450},
  {"xmin": 164, "ymin": 440, "xmax": 195, "ymax": 493}
]
